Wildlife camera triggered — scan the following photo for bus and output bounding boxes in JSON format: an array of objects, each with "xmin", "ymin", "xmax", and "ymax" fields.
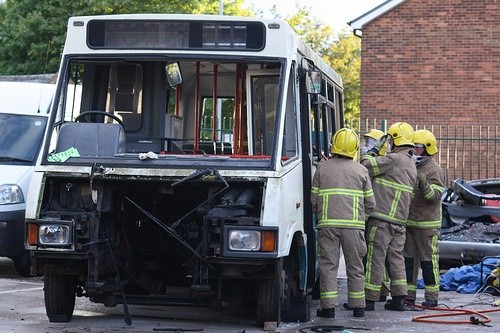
[
  {"xmin": 23, "ymin": 13, "xmax": 347, "ymax": 329},
  {"xmin": 0, "ymin": 80, "xmax": 106, "ymax": 278}
]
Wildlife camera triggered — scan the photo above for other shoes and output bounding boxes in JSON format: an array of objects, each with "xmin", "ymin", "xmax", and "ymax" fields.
[
  {"xmin": 404, "ymin": 294, "xmax": 416, "ymax": 301},
  {"xmin": 379, "ymin": 295, "xmax": 387, "ymax": 301},
  {"xmin": 353, "ymin": 308, "xmax": 364, "ymax": 317},
  {"xmin": 343, "ymin": 297, "xmax": 375, "ymax": 311},
  {"xmin": 317, "ymin": 307, "xmax": 334, "ymax": 318},
  {"xmin": 384, "ymin": 295, "xmax": 405, "ymax": 311},
  {"xmin": 422, "ymin": 298, "xmax": 437, "ymax": 307}
]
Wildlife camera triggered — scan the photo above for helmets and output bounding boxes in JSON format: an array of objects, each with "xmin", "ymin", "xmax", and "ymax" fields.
[
  {"xmin": 331, "ymin": 128, "xmax": 360, "ymax": 158},
  {"xmin": 388, "ymin": 122, "xmax": 415, "ymax": 146},
  {"xmin": 414, "ymin": 129, "xmax": 438, "ymax": 155},
  {"xmin": 364, "ymin": 129, "xmax": 388, "ymax": 155}
]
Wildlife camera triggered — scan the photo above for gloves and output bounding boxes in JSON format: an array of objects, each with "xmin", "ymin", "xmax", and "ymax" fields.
[
  {"xmin": 417, "ymin": 173, "xmax": 428, "ymax": 189},
  {"xmin": 374, "ymin": 134, "xmax": 387, "ymax": 151}
]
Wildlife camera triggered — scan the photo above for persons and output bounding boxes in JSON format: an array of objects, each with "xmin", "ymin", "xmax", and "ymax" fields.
[
  {"xmin": 343, "ymin": 121, "xmax": 447, "ymax": 311},
  {"xmin": 308, "ymin": 126, "xmax": 377, "ymax": 318}
]
